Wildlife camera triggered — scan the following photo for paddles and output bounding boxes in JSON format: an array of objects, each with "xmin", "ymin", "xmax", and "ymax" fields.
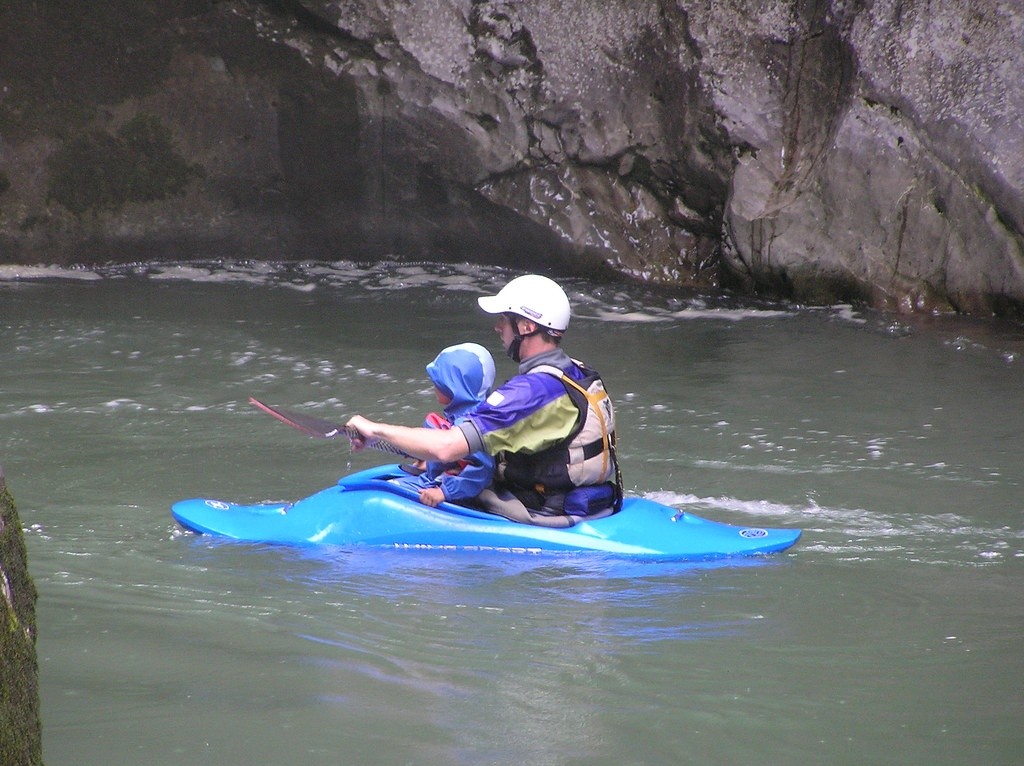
[{"xmin": 247, "ymin": 393, "xmax": 423, "ymax": 464}]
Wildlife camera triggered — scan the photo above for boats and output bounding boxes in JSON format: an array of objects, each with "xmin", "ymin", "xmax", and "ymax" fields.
[{"xmin": 171, "ymin": 462, "xmax": 801, "ymax": 560}]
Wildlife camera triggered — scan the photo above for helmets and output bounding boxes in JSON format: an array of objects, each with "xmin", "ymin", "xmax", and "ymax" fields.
[{"xmin": 477, "ymin": 275, "xmax": 571, "ymax": 332}]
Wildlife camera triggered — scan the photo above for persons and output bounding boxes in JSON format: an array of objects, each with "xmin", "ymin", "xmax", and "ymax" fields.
[
  {"xmin": 391, "ymin": 342, "xmax": 498, "ymax": 509},
  {"xmin": 342, "ymin": 275, "xmax": 617, "ymax": 524}
]
